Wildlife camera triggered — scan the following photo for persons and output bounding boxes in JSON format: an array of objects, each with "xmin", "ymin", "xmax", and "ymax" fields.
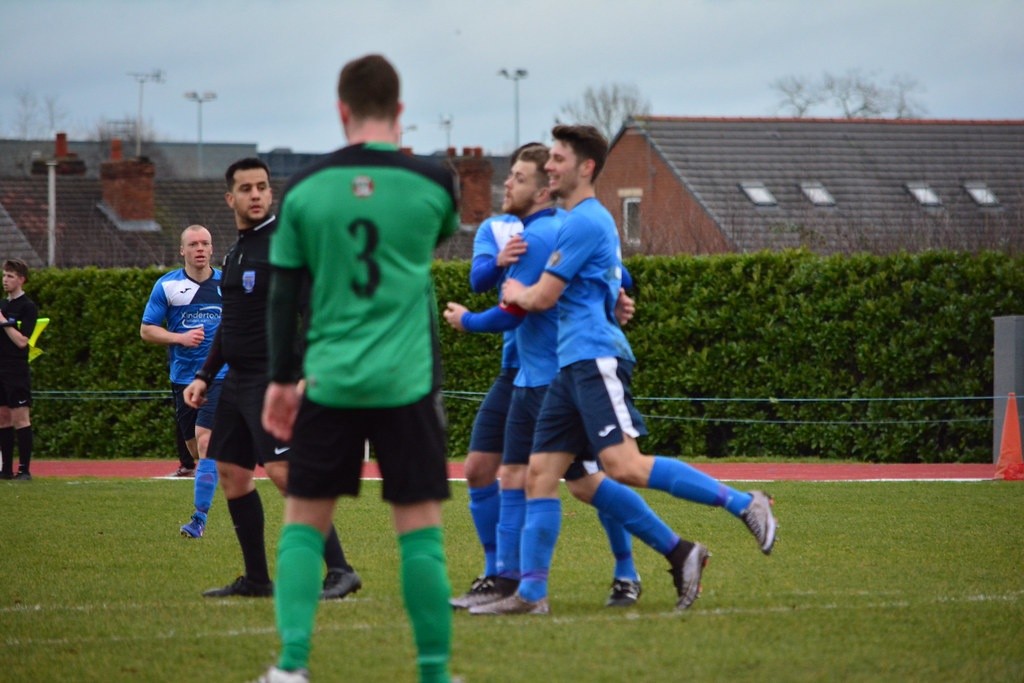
[
  {"xmin": 442, "ymin": 146, "xmax": 712, "ymax": 616},
  {"xmin": 468, "ymin": 125, "xmax": 777, "ymax": 616},
  {"xmin": 140, "ymin": 224, "xmax": 229, "ymax": 538},
  {"xmin": 183, "ymin": 157, "xmax": 363, "ymax": 600},
  {"xmin": 0, "ymin": 256, "xmax": 39, "ymax": 480},
  {"xmin": 258, "ymin": 53, "xmax": 458, "ymax": 683},
  {"xmin": 469, "ymin": 143, "xmax": 643, "ymax": 608}
]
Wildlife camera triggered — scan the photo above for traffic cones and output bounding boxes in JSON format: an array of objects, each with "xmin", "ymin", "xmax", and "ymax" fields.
[{"xmin": 993, "ymin": 392, "xmax": 1024, "ymax": 479}]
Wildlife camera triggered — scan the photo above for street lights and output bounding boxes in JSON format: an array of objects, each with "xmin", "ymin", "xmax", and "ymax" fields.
[
  {"xmin": 496, "ymin": 65, "xmax": 529, "ymax": 152},
  {"xmin": 184, "ymin": 90, "xmax": 218, "ymax": 177}
]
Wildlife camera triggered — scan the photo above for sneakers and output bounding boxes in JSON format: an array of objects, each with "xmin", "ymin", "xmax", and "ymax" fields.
[
  {"xmin": 244, "ymin": 664, "xmax": 310, "ymax": 683},
  {"xmin": 606, "ymin": 576, "xmax": 642, "ymax": 609},
  {"xmin": 741, "ymin": 490, "xmax": 780, "ymax": 555},
  {"xmin": 448, "ymin": 580, "xmax": 519, "ymax": 609},
  {"xmin": 0, "ymin": 470, "xmax": 15, "ymax": 479},
  {"xmin": 461, "ymin": 575, "xmax": 497, "ymax": 594},
  {"xmin": 200, "ymin": 576, "xmax": 275, "ymax": 600},
  {"xmin": 169, "ymin": 462, "xmax": 197, "ymax": 477},
  {"xmin": 179, "ymin": 515, "xmax": 206, "ymax": 539},
  {"xmin": 468, "ymin": 593, "xmax": 552, "ymax": 616},
  {"xmin": 317, "ymin": 563, "xmax": 363, "ymax": 601},
  {"xmin": 665, "ymin": 540, "xmax": 712, "ymax": 613},
  {"xmin": 12, "ymin": 473, "xmax": 32, "ymax": 482}
]
